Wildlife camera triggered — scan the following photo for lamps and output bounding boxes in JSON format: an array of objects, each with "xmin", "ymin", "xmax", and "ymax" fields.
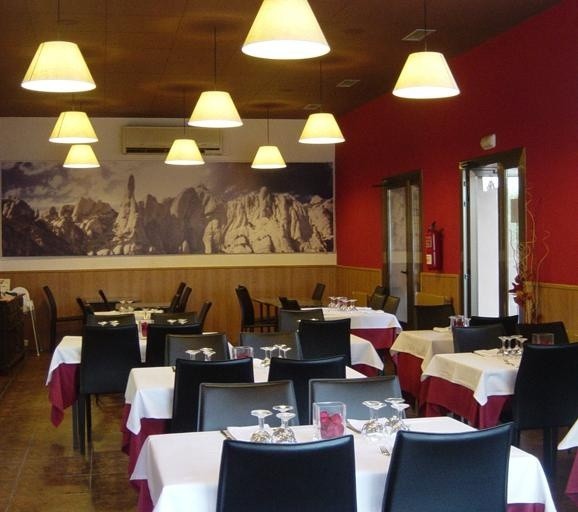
[
  {"xmin": 390, "ymin": 0, "xmax": 464, "ymax": 100},
  {"xmin": 61, "ymin": 143, "xmax": 100, "ymax": 169},
  {"xmin": 298, "ymin": 57, "xmax": 346, "ymax": 147},
  {"xmin": 250, "ymin": 105, "xmax": 287, "ymax": 169},
  {"xmin": 162, "ymin": 86, "xmax": 206, "ymax": 165},
  {"xmin": 47, "ymin": 92, "xmax": 102, "ymax": 147},
  {"xmin": 239, "ymin": 0, "xmax": 331, "ymax": 63},
  {"xmin": 18, "ymin": 0, "xmax": 98, "ymax": 95},
  {"xmin": 185, "ymin": 27, "xmax": 244, "ymax": 127}
]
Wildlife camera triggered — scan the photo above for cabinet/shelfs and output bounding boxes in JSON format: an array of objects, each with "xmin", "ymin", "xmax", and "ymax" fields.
[{"xmin": 0, "ymin": 287, "xmax": 26, "ymax": 376}]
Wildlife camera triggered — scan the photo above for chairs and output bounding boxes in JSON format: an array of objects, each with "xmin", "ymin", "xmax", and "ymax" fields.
[
  {"xmin": 11, "ymin": 286, "xmax": 42, "ymax": 356},
  {"xmin": 197, "ymin": 375, "xmax": 406, "ymax": 432},
  {"xmin": 46, "ymin": 313, "xmax": 239, "ymax": 457},
  {"xmin": 381, "ymin": 421, "xmax": 513, "ymax": 511},
  {"xmin": 236, "ymin": 283, "xmax": 400, "ymax": 373},
  {"xmin": 216, "ymin": 434, "xmax": 357, "ymax": 511},
  {"xmin": 43, "ymin": 282, "xmax": 213, "ymax": 352},
  {"xmin": 390, "ymin": 292, "xmax": 577, "ymax": 511}
]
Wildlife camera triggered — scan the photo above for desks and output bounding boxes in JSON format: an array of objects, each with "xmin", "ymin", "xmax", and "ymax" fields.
[{"xmin": 129, "ymin": 415, "xmax": 556, "ymax": 512}]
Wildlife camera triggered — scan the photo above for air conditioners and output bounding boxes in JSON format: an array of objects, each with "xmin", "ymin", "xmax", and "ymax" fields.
[{"xmin": 118, "ymin": 124, "xmax": 224, "ymax": 157}]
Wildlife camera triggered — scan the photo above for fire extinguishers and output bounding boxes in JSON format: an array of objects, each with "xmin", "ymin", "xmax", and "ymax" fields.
[{"xmin": 423, "ymin": 221, "xmax": 442, "ymax": 269}]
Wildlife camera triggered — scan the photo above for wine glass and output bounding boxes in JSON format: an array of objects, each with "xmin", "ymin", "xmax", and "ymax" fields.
[
  {"xmin": 249, "ymin": 398, "xmax": 411, "ymax": 449},
  {"xmin": 448, "ymin": 314, "xmax": 528, "ymax": 362},
  {"xmin": 328, "ymin": 295, "xmax": 363, "ymax": 315},
  {"xmin": 96, "ymin": 298, "xmax": 294, "ymax": 376}
]
